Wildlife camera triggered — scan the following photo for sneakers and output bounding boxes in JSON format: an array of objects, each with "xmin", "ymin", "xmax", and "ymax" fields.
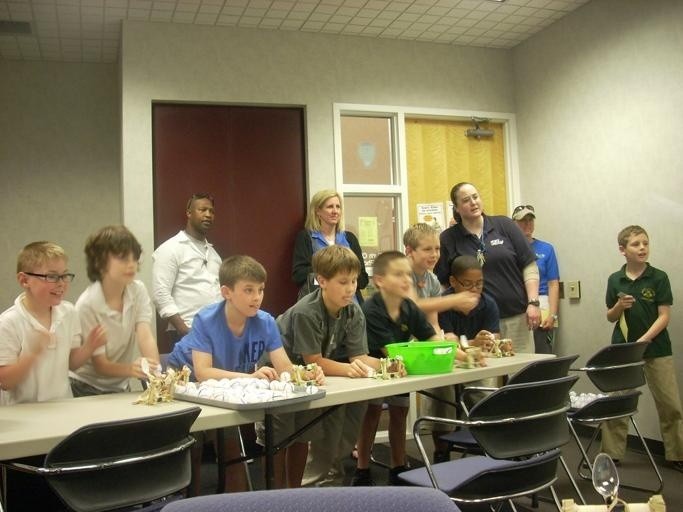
[
  {"xmin": 351, "ymin": 445, "xmax": 452, "ymax": 485},
  {"xmin": 589, "ymin": 458, "xmax": 620, "ymax": 468},
  {"xmin": 670, "ymin": 459, "xmax": 682, "ymax": 472}
]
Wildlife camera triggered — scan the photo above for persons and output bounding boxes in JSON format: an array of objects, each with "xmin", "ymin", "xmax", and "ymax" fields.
[
  {"xmin": 0, "ymin": 241, "xmax": 108, "ymax": 512},
  {"xmin": 403, "ymin": 183, "xmax": 561, "ymax": 463},
  {"xmin": 254, "ymin": 246, "xmax": 407, "ymax": 488},
  {"xmin": 152, "ymin": 191, "xmax": 223, "ymax": 352},
  {"xmin": 168, "ymin": 255, "xmax": 326, "ymax": 496},
  {"xmin": 290, "ymin": 188, "xmax": 369, "ymax": 304},
  {"xmin": 350, "ymin": 251, "xmax": 487, "ymax": 488},
  {"xmin": 583, "ymin": 226, "xmax": 683, "ymax": 472},
  {"xmin": 68, "ymin": 224, "xmax": 160, "ymax": 398}
]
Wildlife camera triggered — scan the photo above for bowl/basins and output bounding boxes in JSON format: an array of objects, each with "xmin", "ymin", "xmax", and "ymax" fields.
[{"xmin": 384, "ymin": 341, "xmax": 457, "ymax": 375}]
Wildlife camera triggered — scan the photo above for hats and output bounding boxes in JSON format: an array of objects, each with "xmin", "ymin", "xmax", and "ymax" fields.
[{"xmin": 513, "ymin": 206, "xmax": 536, "ymax": 221}]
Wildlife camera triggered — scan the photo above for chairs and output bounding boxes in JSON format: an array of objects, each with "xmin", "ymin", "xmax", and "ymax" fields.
[
  {"xmin": 397, "ymin": 370, "xmax": 581, "ymax": 512},
  {"xmin": 1, "ymin": 405, "xmax": 202, "ymax": 512},
  {"xmin": 159, "ymin": 486, "xmax": 464, "ymax": 512},
  {"xmin": 564, "ymin": 338, "xmax": 663, "ymax": 503},
  {"xmin": 437, "ymin": 350, "xmax": 588, "ymax": 512}
]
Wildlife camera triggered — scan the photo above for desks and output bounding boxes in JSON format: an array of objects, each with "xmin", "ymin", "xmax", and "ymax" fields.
[
  {"xmin": 1, "ymin": 391, "xmax": 264, "ymax": 490},
  {"xmin": 216, "ymin": 352, "xmax": 559, "ymax": 497}
]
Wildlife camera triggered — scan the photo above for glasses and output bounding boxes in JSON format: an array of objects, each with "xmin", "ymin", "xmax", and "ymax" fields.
[
  {"xmin": 454, "ymin": 277, "xmax": 485, "ymax": 290},
  {"xmin": 512, "ymin": 205, "xmax": 534, "ymax": 218},
  {"xmin": 23, "ymin": 272, "xmax": 75, "ymax": 283},
  {"xmin": 188, "ymin": 193, "xmax": 216, "ymax": 210}
]
